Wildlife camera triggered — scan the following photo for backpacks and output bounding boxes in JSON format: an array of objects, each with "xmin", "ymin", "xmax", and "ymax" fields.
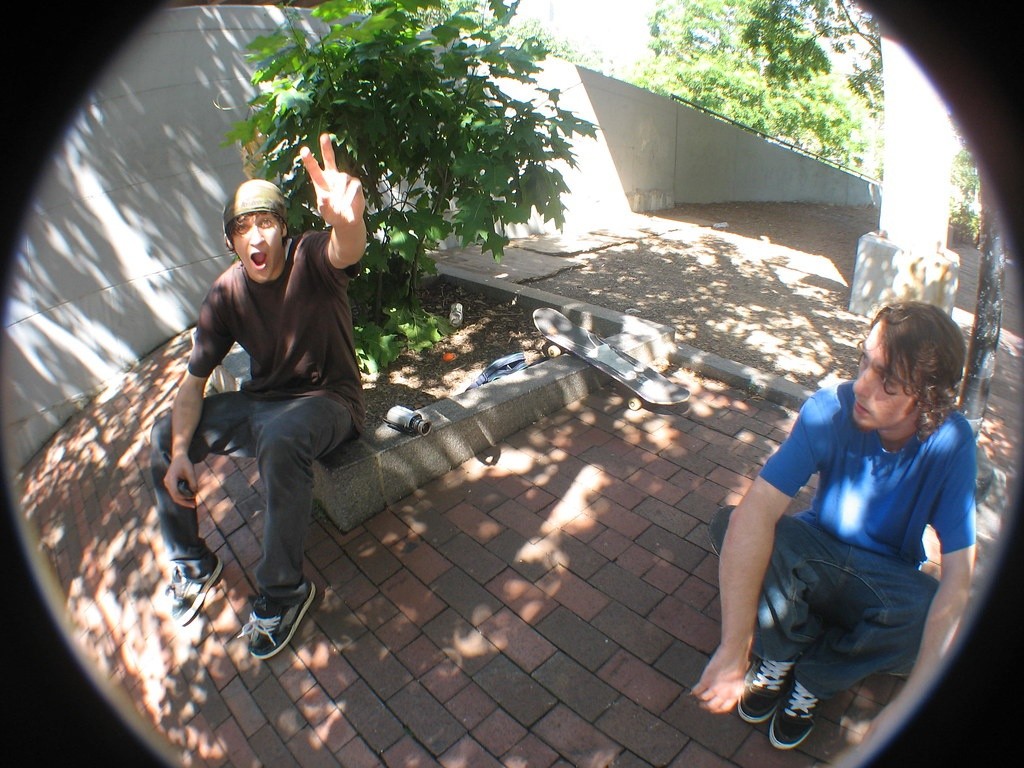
[{"xmin": 464, "ymin": 349, "xmax": 548, "ymax": 393}]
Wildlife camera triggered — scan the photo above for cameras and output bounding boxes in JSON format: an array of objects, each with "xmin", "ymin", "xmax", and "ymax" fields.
[{"xmin": 386, "ymin": 404, "xmax": 432, "ymax": 437}]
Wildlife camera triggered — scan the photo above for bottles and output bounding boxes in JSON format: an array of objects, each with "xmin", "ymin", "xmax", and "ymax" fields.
[
  {"xmin": 383, "ymin": 405, "xmax": 432, "ymax": 436},
  {"xmin": 449, "ymin": 303, "xmax": 463, "ymax": 327}
]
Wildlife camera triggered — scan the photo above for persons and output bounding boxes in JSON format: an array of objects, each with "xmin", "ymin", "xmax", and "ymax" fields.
[
  {"xmin": 690, "ymin": 302, "xmax": 978, "ymax": 768},
  {"xmin": 149, "ymin": 131, "xmax": 366, "ymax": 659}
]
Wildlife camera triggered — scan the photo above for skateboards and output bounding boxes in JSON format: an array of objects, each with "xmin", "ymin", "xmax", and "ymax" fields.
[{"xmin": 534, "ymin": 306, "xmax": 690, "ymax": 411}]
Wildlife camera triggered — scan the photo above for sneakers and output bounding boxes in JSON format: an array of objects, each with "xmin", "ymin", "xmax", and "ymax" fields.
[
  {"xmin": 735, "ymin": 659, "xmax": 799, "ymax": 724},
  {"xmin": 767, "ymin": 677, "xmax": 829, "ymax": 751},
  {"xmin": 238, "ymin": 582, "xmax": 317, "ymax": 660},
  {"xmin": 164, "ymin": 555, "xmax": 224, "ymax": 627}
]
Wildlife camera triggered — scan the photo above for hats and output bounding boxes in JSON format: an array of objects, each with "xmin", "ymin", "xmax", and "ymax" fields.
[{"xmin": 220, "ymin": 179, "xmax": 289, "ymax": 228}]
